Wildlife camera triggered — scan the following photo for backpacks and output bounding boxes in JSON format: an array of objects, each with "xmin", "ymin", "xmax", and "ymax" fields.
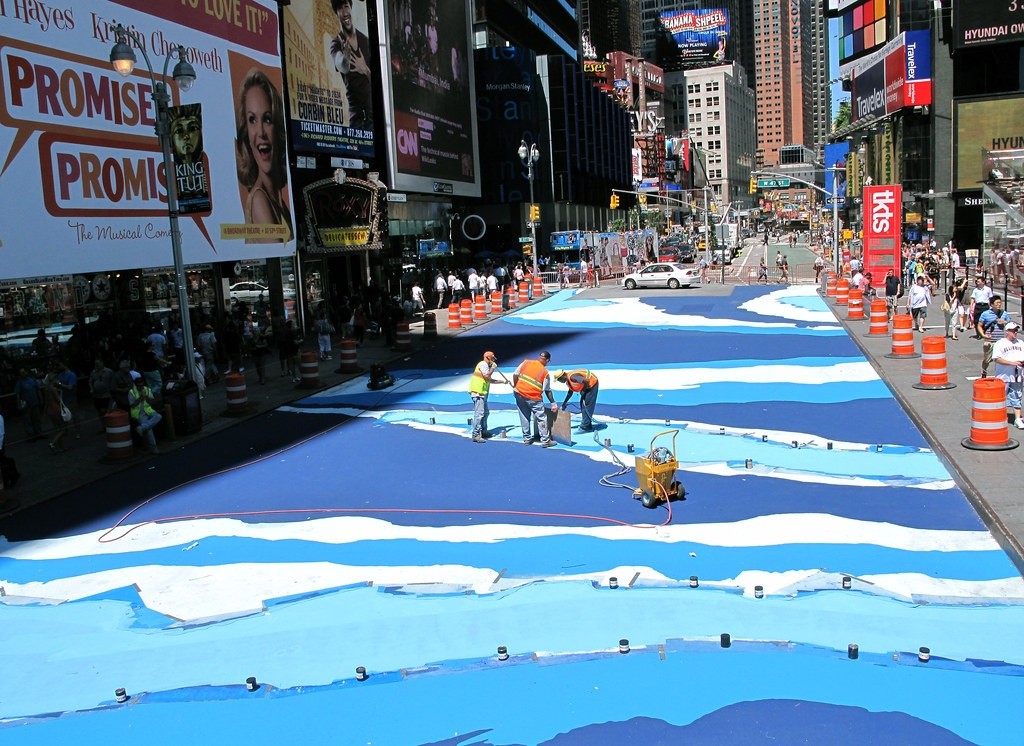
[{"xmin": 897, "ymin": 283, "xmax": 904, "ymax": 297}]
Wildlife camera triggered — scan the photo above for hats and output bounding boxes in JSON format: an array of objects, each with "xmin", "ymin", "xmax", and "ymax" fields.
[
  {"xmin": 554, "ymin": 369, "xmax": 564, "ymax": 381},
  {"xmin": 484, "ymin": 352, "xmax": 497, "ymax": 361},
  {"xmin": 540, "ymin": 351, "xmax": 552, "ymax": 364},
  {"xmin": 1004, "ymin": 322, "xmax": 1020, "ymax": 330}
]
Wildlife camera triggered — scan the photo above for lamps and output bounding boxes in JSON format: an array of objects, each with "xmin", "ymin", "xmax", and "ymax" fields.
[{"xmin": 824, "ymin": 73, "xmax": 851, "ymax": 87}]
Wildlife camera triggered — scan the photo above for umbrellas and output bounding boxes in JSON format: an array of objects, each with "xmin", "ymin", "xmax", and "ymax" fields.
[{"xmin": 474, "ymin": 248, "xmax": 520, "ymax": 266}]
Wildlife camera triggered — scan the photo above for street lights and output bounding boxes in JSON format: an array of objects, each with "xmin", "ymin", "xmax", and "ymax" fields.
[
  {"xmin": 107, "ymin": 21, "xmax": 198, "ymax": 388},
  {"xmin": 518, "ymin": 139, "xmax": 540, "ymax": 276}
]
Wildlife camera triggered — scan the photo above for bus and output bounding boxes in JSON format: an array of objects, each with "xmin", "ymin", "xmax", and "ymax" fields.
[{"xmin": 549, "ymin": 229, "xmax": 660, "ymax": 281}]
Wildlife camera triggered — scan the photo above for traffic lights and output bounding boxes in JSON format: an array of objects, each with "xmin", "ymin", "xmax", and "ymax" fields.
[
  {"xmin": 749, "ymin": 176, "xmax": 757, "ymax": 196},
  {"xmin": 529, "ymin": 205, "xmax": 539, "ymax": 221},
  {"xmin": 610, "ymin": 194, "xmax": 619, "ymax": 210}
]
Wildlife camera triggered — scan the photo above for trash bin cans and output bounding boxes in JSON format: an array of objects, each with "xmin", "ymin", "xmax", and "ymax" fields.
[{"xmin": 169, "ymin": 379, "xmax": 203, "ymax": 436}]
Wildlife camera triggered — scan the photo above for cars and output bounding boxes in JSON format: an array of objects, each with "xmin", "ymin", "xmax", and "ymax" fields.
[
  {"xmin": 228, "ymin": 280, "xmax": 296, "ymax": 301},
  {"xmin": 620, "ymin": 263, "xmax": 700, "ymax": 289},
  {"xmin": 659, "ymin": 222, "xmax": 784, "ymax": 264}
]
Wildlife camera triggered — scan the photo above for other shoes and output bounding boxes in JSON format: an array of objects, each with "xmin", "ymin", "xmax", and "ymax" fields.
[
  {"xmin": 981, "ymin": 373, "xmax": 986, "ymax": 378},
  {"xmin": 913, "ymin": 326, "xmax": 918, "ymax": 329},
  {"xmin": 960, "ymin": 327, "xmax": 963, "ymax": 332},
  {"xmin": 919, "ymin": 327, "xmax": 924, "ymax": 333},
  {"xmin": 946, "ymin": 331, "xmax": 948, "ymax": 336},
  {"xmin": 952, "ymin": 337, "xmax": 958, "ymax": 340},
  {"xmin": 473, "ymin": 436, "xmax": 486, "ymax": 443},
  {"xmin": 890, "ymin": 318, "xmax": 893, "ymax": 320},
  {"xmin": 969, "ymin": 326, "xmax": 973, "ymax": 329},
  {"xmin": 525, "ymin": 440, "xmax": 533, "ymax": 446},
  {"xmin": 978, "ymin": 336, "xmax": 981, "ymax": 340},
  {"xmin": 542, "ymin": 440, "xmax": 557, "ymax": 448},
  {"xmin": 482, "ymin": 432, "xmax": 491, "ymax": 438}
]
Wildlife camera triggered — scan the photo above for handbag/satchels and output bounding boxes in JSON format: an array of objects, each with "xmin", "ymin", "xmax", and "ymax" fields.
[
  {"xmin": 813, "ymin": 263, "xmax": 816, "ymax": 270},
  {"xmin": 958, "ymin": 305, "xmax": 964, "ymax": 315},
  {"xmin": 941, "ymin": 301, "xmax": 950, "ymax": 312}
]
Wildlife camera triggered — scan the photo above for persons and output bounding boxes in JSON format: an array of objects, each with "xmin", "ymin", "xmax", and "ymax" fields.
[
  {"xmin": 977, "ymin": 295, "xmax": 1009, "ymax": 378},
  {"xmin": 991, "ymin": 322, "xmax": 1024, "ymax": 429},
  {"xmin": 280, "ymin": 267, "xmax": 426, "ymax": 383},
  {"xmin": 643, "ymin": 234, "xmax": 655, "ymax": 260},
  {"xmin": 236, "ymin": 67, "xmax": 293, "ymax": 243},
  {"xmin": 31, "ymin": 276, "xmax": 271, "ymax": 373},
  {"xmin": 758, "ymin": 230, "xmax": 992, "ymax": 340},
  {"xmin": 329, "ymin": 0, "xmax": 373, "ymax": 132},
  {"xmin": 990, "ymin": 239, "xmax": 1024, "ymax": 284},
  {"xmin": 431, "ymin": 254, "xmax": 593, "ymax": 309},
  {"xmin": 513, "ymin": 350, "xmax": 558, "ymax": 449},
  {"xmin": 713, "ymin": 37, "xmax": 726, "ymax": 64},
  {"xmin": 0, "ymin": 316, "xmax": 266, "ymax": 493},
  {"xmin": 698, "ymin": 257, "xmax": 710, "ymax": 284},
  {"xmin": 553, "ymin": 369, "xmax": 599, "ymax": 435},
  {"xmin": 468, "ymin": 351, "xmax": 509, "ymax": 443}
]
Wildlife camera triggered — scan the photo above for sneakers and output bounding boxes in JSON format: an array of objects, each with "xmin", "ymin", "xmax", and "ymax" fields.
[{"xmin": 1014, "ymin": 418, "xmax": 1024, "ymax": 429}]
